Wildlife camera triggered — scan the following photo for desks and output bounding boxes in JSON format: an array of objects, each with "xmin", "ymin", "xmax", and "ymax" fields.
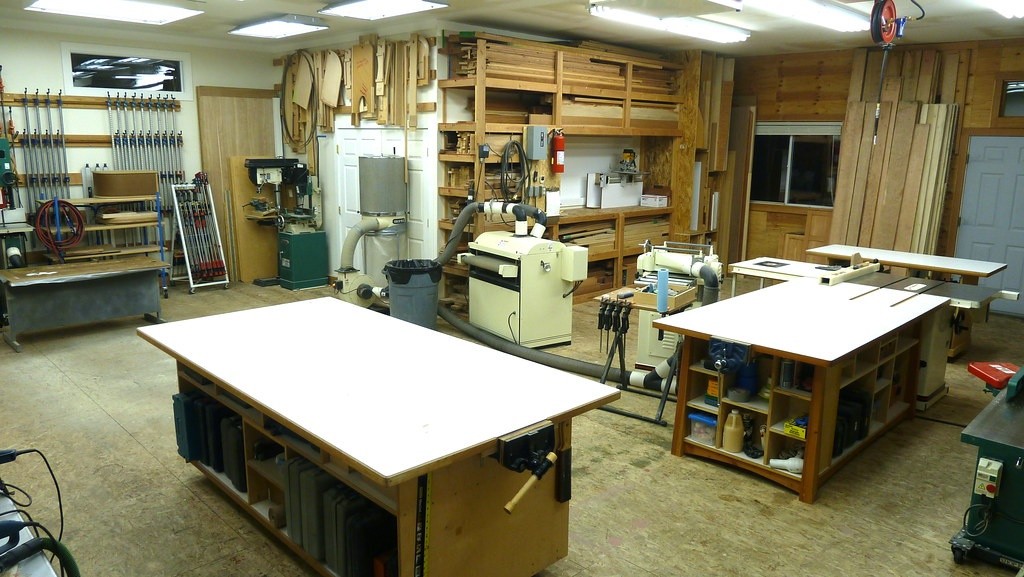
[
  {"xmin": 135, "ymin": 294, "xmax": 621, "ymax": 577},
  {"xmin": 728, "ymin": 255, "xmax": 1003, "ymax": 412},
  {"xmin": 806, "ymin": 243, "xmax": 1008, "ymax": 357},
  {"xmin": 0, "ymin": 256, "xmax": 170, "ymax": 353},
  {"xmin": 652, "ymin": 274, "xmax": 952, "ymax": 505}
]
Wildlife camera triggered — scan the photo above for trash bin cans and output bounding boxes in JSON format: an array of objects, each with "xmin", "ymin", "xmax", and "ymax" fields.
[{"xmin": 384, "ymin": 258, "xmax": 441, "ymax": 329}]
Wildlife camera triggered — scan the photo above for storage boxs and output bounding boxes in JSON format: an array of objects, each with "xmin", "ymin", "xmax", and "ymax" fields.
[
  {"xmin": 783, "ymin": 412, "xmax": 809, "ymax": 439},
  {"xmin": 641, "ymin": 194, "xmax": 668, "ymax": 207},
  {"xmin": 688, "ymin": 413, "xmax": 717, "ymax": 446},
  {"xmin": 171, "ymin": 389, "xmax": 399, "ymax": 576},
  {"xmin": 574, "ymin": 263, "xmax": 637, "ymax": 295},
  {"xmin": 634, "ymin": 282, "xmax": 696, "ymax": 310}
]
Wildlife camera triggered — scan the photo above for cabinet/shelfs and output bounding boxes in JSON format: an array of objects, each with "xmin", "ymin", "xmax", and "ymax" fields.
[
  {"xmin": 437, "ymin": 29, "xmax": 683, "ymax": 314},
  {"xmin": 35, "ymin": 197, "xmax": 168, "ymax": 261}
]
